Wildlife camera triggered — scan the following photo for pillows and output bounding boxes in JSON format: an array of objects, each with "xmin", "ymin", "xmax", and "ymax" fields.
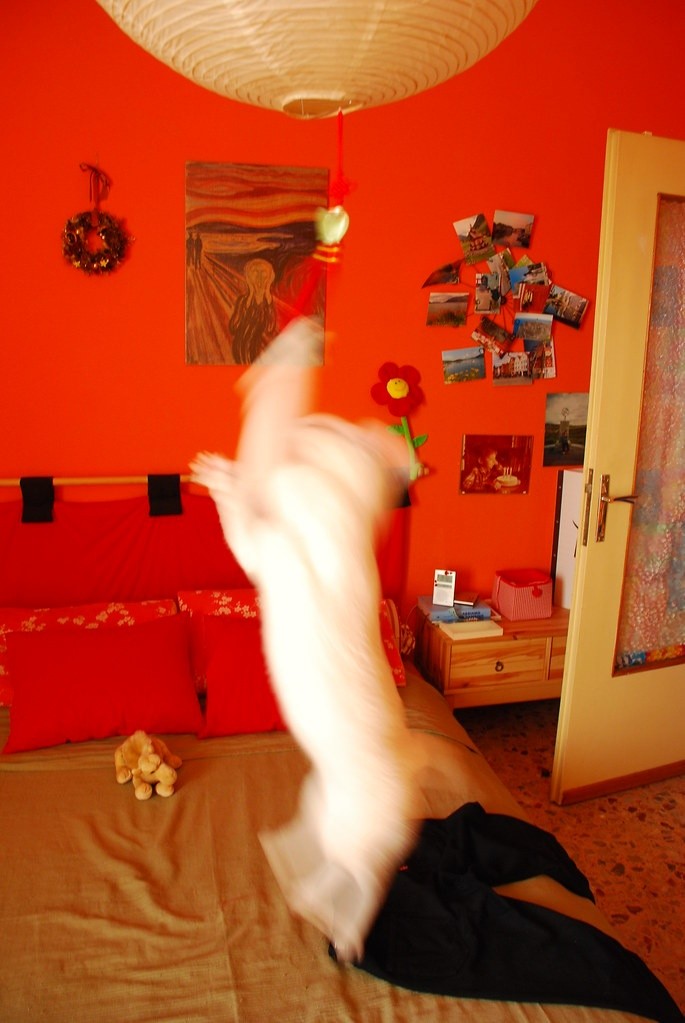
[
  {"xmin": 177, "ymin": 587, "xmax": 407, "ymax": 740},
  {"xmin": 0, "ymin": 598, "xmax": 203, "ymax": 756}
]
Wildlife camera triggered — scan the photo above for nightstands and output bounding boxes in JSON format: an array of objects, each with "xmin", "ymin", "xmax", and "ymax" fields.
[{"xmin": 423, "ymin": 605, "xmax": 570, "ymax": 714}]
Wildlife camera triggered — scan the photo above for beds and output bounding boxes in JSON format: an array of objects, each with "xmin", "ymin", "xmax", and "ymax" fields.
[{"xmin": 0, "ymin": 474, "xmax": 676, "ymax": 1023}]
